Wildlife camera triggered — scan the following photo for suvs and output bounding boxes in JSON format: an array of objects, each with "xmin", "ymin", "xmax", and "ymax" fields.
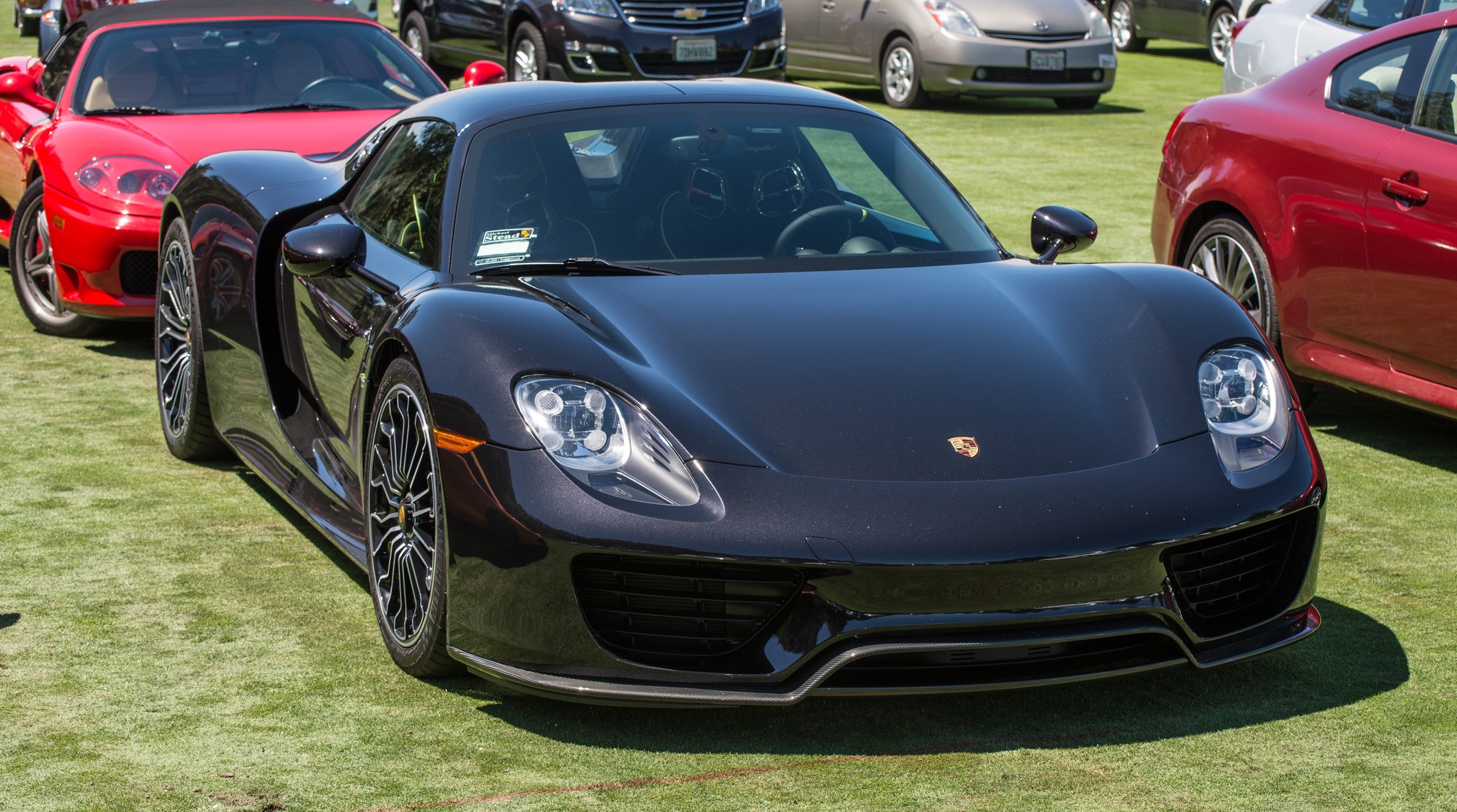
[
  {"xmin": 396, "ymin": 0, "xmax": 788, "ymax": 81},
  {"xmin": 781, "ymin": 0, "xmax": 1117, "ymax": 113}
]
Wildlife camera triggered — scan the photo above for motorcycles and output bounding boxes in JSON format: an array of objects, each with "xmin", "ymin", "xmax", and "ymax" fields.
[{"xmin": 13, "ymin": 0, "xmax": 159, "ymax": 57}]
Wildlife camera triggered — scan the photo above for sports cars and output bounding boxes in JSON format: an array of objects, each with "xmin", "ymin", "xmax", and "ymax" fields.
[
  {"xmin": 0, "ymin": 0, "xmax": 452, "ymax": 338},
  {"xmin": 154, "ymin": 79, "xmax": 1330, "ymax": 716}
]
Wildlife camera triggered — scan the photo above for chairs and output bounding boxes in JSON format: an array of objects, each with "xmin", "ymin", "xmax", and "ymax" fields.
[
  {"xmin": 84, "ymin": 49, "xmax": 176, "ymax": 111},
  {"xmin": 252, "ymin": 43, "xmax": 324, "ymax": 105}
]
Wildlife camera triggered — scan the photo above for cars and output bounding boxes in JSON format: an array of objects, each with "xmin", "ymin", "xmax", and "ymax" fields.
[
  {"xmin": 1150, "ymin": 9, "xmax": 1457, "ymax": 421},
  {"xmin": 1221, "ymin": 0, "xmax": 1457, "ymax": 134},
  {"xmin": 1087, "ymin": 0, "xmax": 1272, "ymax": 66}
]
